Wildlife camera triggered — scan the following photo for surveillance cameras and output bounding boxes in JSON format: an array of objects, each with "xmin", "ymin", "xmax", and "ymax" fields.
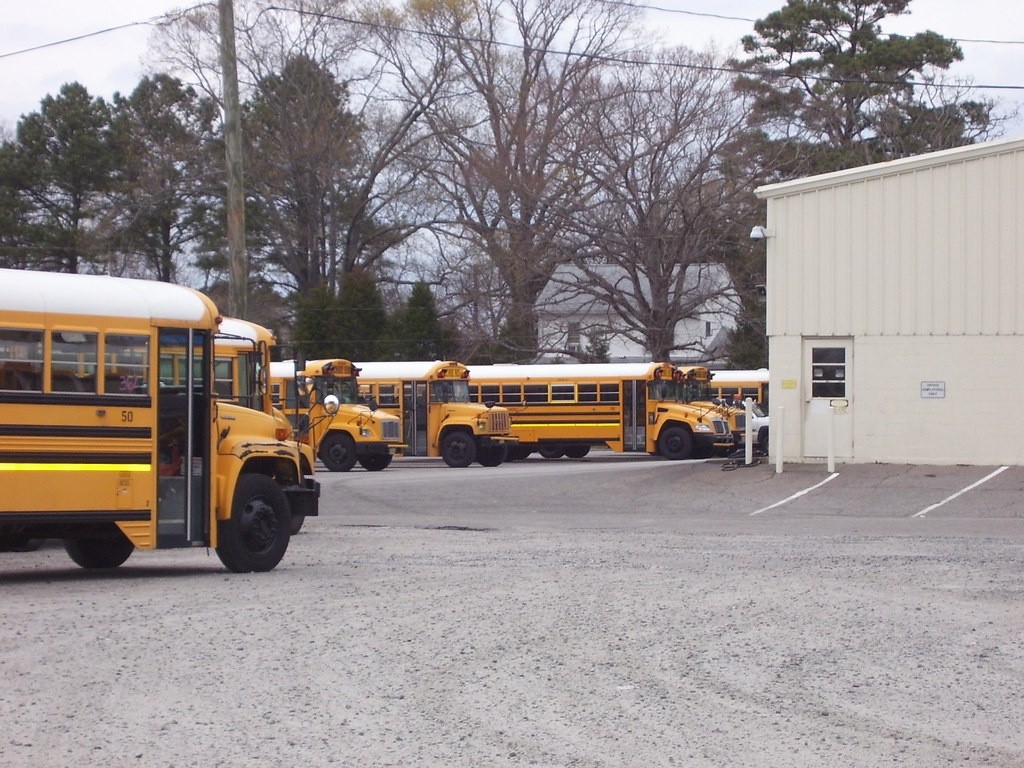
[{"xmin": 750, "ymin": 226, "xmax": 764, "ymax": 242}]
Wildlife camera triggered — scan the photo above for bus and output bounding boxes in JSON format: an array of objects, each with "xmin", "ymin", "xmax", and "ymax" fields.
[
  {"xmin": 336, "ymin": 360, "xmax": 522, "ymax": 468},
  {"xmin": 449, "ymin": 363, "xmax": 770, "ymax": 462},
  {"xmin": 271, "ymin": 361, "xmax": 411, "ymax": 473},
  {"xmin": 0, "ymin": 270, "xmax": 340, "ymax": 578}
]
